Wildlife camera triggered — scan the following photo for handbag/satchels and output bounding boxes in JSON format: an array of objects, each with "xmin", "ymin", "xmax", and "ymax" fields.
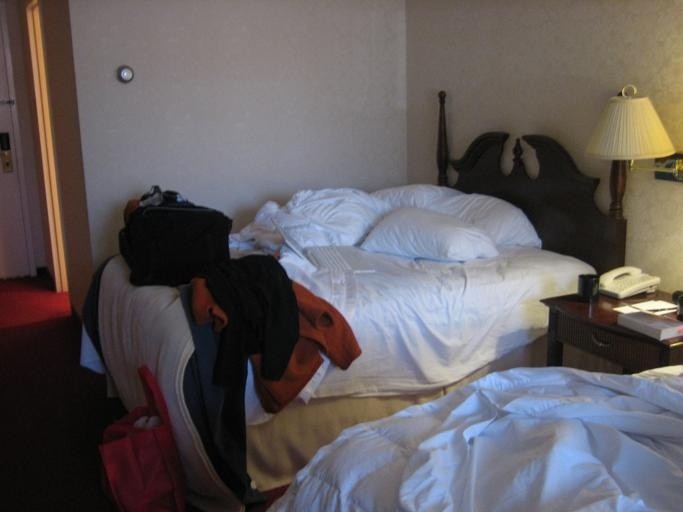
[
  {"xmin": 99, "ymin": 365, "xmax": 187, "ymax": 512},
  {"xmin": 118, "ymin": 185, "xmax": 233, "ymax": 287}
]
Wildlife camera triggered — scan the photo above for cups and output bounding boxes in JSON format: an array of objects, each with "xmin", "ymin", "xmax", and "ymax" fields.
[{"xmin": 576, "ymin": 274, "xmax": 600, "ymax": 302}]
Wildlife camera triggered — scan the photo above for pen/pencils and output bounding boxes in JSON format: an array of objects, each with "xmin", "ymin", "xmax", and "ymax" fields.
[{"xmin": 647, "ymin": 308, "xmax": 677, "ymax": 312}]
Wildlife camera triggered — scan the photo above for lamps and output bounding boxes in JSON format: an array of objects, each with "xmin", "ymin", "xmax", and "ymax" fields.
[{"xmin": 583, "ymin": 83, "xmax": 683, "ymax": 182}]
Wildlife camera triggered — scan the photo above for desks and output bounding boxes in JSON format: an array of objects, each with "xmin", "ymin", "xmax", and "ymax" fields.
[{"xmin": 540, "ymin": 288, "xmax": 683, "ymax": 376}]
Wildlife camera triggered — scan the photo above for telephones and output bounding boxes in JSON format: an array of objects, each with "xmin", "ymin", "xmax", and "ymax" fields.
[{"xmin": 599, "ymin": 266, "xmax": 661, "ymax": 300}]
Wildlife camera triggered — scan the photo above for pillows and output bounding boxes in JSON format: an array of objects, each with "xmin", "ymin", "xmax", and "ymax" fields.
[
  {"xmin": 371, "ymin": 183, "xmax": 543, "ymax": 248},
  {"xmin": 357, "ymin": 208, "xmax": 498, "ymax": 262}
]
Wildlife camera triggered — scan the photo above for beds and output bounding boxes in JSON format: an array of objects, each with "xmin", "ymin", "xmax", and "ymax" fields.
[
  {"xmin": 267, "ymin": 365, "xmax": 683, "ymax": 512},
  {"xmin": 79, "ymin": 89, "xmax": 631, "ymax": 512}
]
